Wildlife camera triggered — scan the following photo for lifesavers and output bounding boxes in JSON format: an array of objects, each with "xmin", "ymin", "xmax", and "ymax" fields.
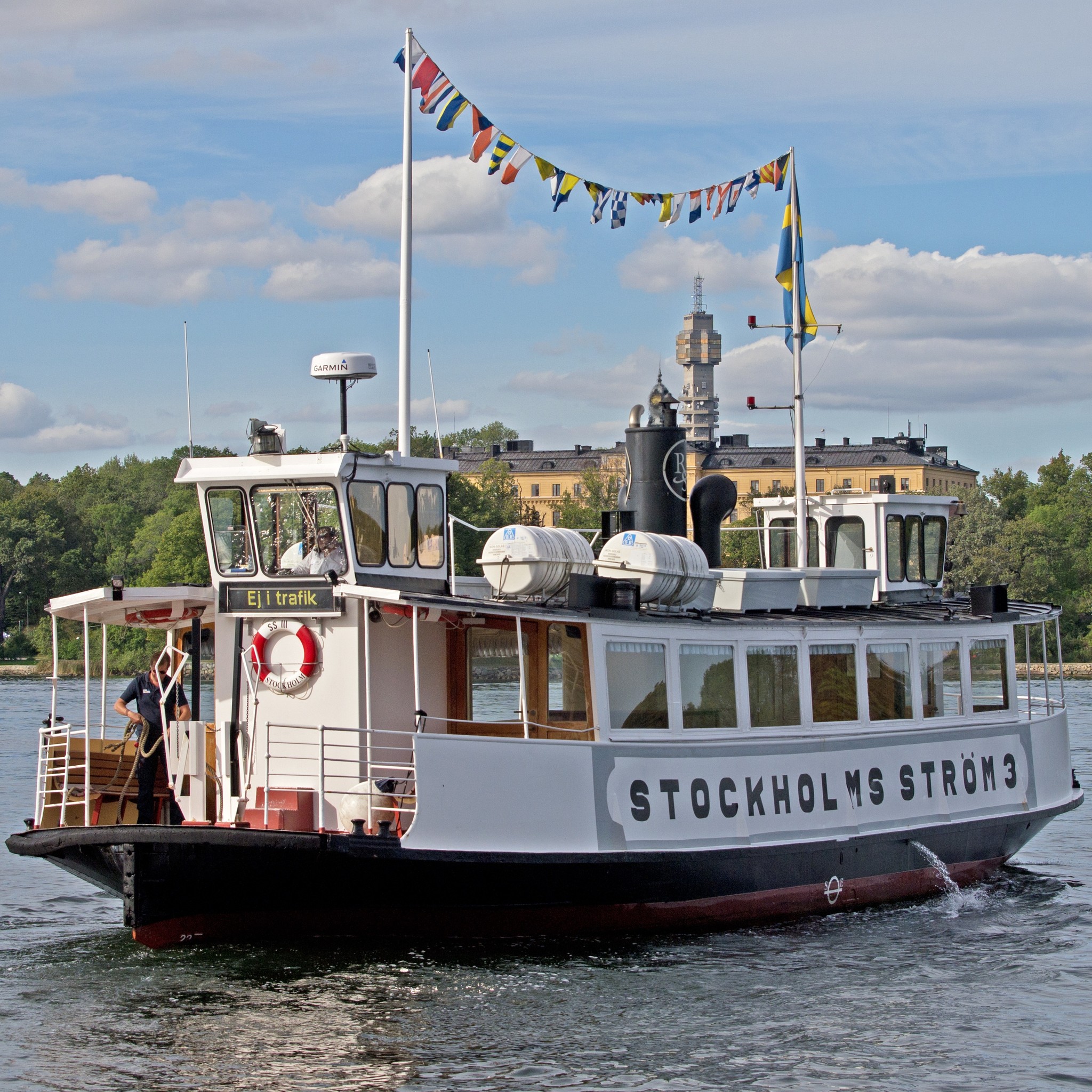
[
  {"xmin": 249, "ymin": 617, "xmax": 319, "ymax": 696},
  {"xmin": 126, "ymin": 608, "xmax": 198, "ymax": 624},
  {"xmin": 382, "ymin": 603, "xmax": 458, "ymax": 622}
]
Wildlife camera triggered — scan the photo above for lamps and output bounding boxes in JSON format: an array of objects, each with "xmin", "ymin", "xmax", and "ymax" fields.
[{"xmin": 111, "ymin": 575, "xmax": 124, "ymax": 591}]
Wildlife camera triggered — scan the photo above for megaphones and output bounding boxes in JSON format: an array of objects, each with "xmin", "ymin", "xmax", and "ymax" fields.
[{"xmin": 369, "ymin": 606, "xmax": 382, "ymax": 623}]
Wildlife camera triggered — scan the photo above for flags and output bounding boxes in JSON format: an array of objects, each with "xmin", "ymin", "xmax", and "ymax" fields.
[
  {"xmin": 689, "ymin": 189, "xmax": 702, "ymax": 224},
  {"xmin": 664, "ymin": 193, "xmax": 686, "ymax": 229},
  {"xmin": 774, "ymin": 153, "xmax": 790, "ymax": 192},
  {"xmin": 629, "ymin": 192, "xmax": 664, "ymax": 206},
  {"xmin": 392, "ymin": 36, "xmax": 424, "ymax": 73},
  {"xmin": 436, "ymin": 90, "xmax": 468, "ymax": 131},
  {"xmin": 611, "ymin": 190, "xmax": 628, "ymax": 229},
  {"xmin": 590, "ymin": 186, "xmax": 612, "ymax": 224},
  {"xmin": 552, "ymin": 172, "xmax": 579, "ymax": 212},
  {"xmin": 712, "ymin": 180, "xmax": 732, "ymax": 221},
  {"xmin": 501, "ymin": 145, "xmax": 532, "ymax": 185},
  {"xmin": 487, "ymin": 133, "xmax": 515, "ymax": 175},
  {"xmin": 659, "ymin": 192, "xmax": 674, "ymax": 222},
  {"xmin": 742, "ymin": 168, "xmax": 760, "ymax": 199},
  {"xmin": 726, "ymin": 176, "xmax": 746, "ymax": 214},
  {"xmin": 759, "ymin": 161, "xmax": 775, "ymax": 185},
  {"xmin": 412, "ymin": 55, "xmax": 440, "ymax": 99},
  {"xmin": 705, "ymin": 185, "xmax": 716, "ymax": 210},
  {"xmin": 775, "ymin": 170, "xmax": 818, "ymax": 355},
  {"xmin": 418, "ymin": 72, "xmax": 455, "ymax": 114},
  {"xmin": 469, "ymin": 125, "xmax": 500, "ymax": 163},
  {"xmin": 550, "ymin": 166, "xmax": 565, "ymax": 202},
  {"xmin": 472, "ymin": 104, "xmax": 493, "ymax": 137},
  {"xmin": 583, "ymin": 180, "xmax": 605, "ymax": 202},
  {"xmin": 534, "ymin": 155, "xmax": 555, "ymax": 181}
]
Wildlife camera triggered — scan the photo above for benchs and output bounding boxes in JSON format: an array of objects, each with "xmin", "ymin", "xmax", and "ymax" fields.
[{"xmin": 53, "ymin": 750, "xmax": 170, "ymax": 826}]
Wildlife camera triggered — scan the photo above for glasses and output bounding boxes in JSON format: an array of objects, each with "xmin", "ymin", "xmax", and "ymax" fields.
[{"xmin": 152, "ymin": 666, "xmax": 167, "ymax": 674}]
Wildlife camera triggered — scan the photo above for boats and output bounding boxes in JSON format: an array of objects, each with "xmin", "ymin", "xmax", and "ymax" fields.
[{"xmin": 7, "ymin": 29, "xmax": 1087, "ymax": 949}]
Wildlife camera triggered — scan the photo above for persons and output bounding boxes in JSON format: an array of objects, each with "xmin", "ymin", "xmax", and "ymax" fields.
[
  {"xmin": 113, "ymin": 651, "xmax": 192, "ymax": 824},
  {"xmin": 290, "ymin": 526, "xmax": 345, "ymax": 576}
]
